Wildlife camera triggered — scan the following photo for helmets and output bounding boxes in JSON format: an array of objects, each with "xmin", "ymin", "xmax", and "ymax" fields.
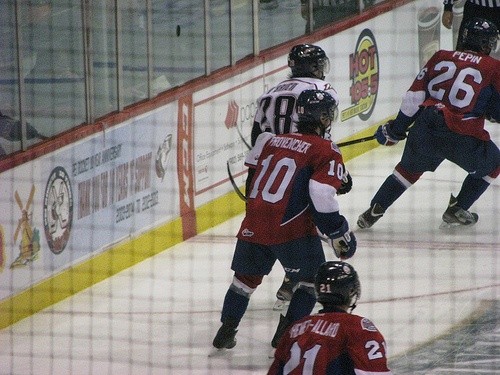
[
  {"xmin": 315, "ymin": 261, "xmax": 361, "ymax": 301},
  {"xmin": 288, "ymin": 44, "xmax": 327, "ymax": 72},
  {"xmin": 457, "ymin": 17, "xmax": 497, "ymax": 48},
  {"xmin": 295, "ymin": 90, "xmax": 336, "ymax": 125}
]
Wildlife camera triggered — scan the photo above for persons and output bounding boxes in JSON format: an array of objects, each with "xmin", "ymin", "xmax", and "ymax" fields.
[
  {"xmin": 205, "ymin": 90, "xmax": 359, "ymax": 360},
  {"xmin": 251, "ymin": 0, "xmax": 500, "ymax": 230},
  {"xmin": 268, "ymin": 261, "xmax": 393, "ymax": 375}
]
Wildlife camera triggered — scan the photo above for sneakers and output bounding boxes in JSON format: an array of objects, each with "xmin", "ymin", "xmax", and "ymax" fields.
[
  {"xmin": 273, "ymin": 281, "xmax": 294, "ymax": 311},
  {"xmin": 439, "ymin": 193, "xmax": 479, "ymax": 228},
  {"xmin": 268, "ymin": 313, "xmax": 292, "ymax": 357},
  {"xmin": 357, "ymin": 202, "xmax": 385, "ymax": 228},
  {"xmin": 207, "ymin": 324, "xmax": 239, "ymax": 358}
]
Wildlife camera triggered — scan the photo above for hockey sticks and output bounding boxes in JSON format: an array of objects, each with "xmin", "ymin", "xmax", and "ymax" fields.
[
  {"xmin": 234, "ymin": 121, "xmax": 252, "ymax": 151},
  {"xmin": 334, "ymin": 127, "xmax": 411, "ymax": 148},
  {"xmin": 226, "ymin": 159, "xmax": 329, "ymax": 245}
]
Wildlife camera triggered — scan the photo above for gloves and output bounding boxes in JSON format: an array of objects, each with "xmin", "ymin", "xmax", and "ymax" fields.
[
  {"xmin": 336, "ymin": 171, "xmax": 352, "ymax": 194},
  {"xmin": 327, "ymin": 214, "xmax": 356, "ymax": 260},
  {"xmin": 377, "ymin": 120, "xmax": 407, "ymax": 146}
]
